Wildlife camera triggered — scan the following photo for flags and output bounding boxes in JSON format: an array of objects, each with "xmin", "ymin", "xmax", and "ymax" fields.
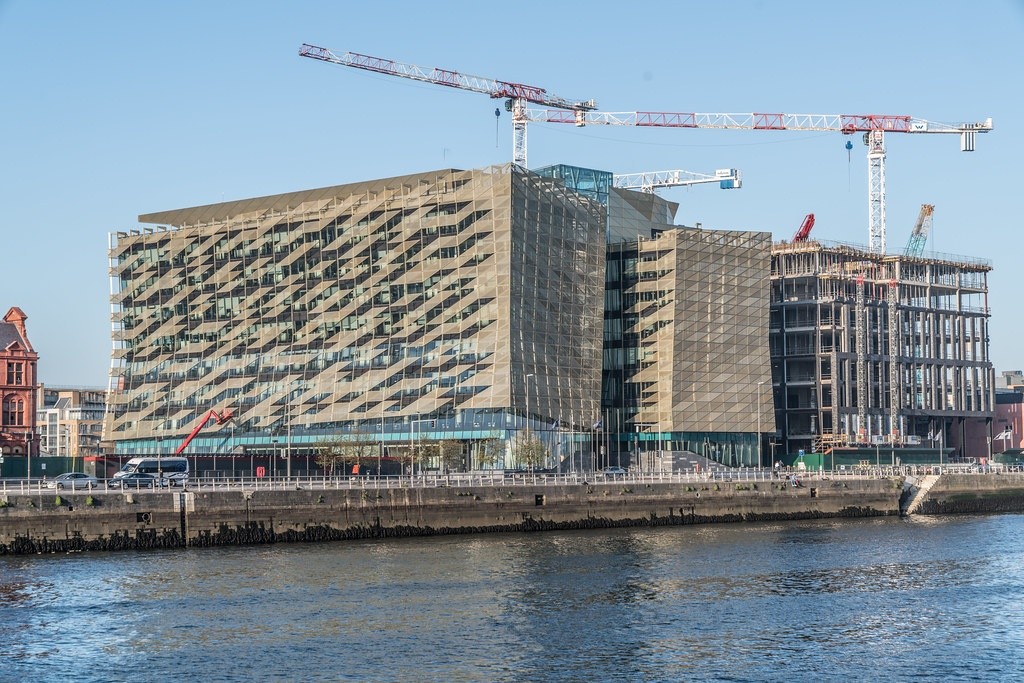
[
  {"xmin": 928, "ymin": 431, "xmax": 933, "ymax": 439},
  {"xmin": 934, "ymin": 432, "xmax": 941, "ymax": 442},
  {"xmin": 1004, "ymin": 432, "xmax": 1011, "ymax": 439},
  {"xmin": 552, "ymin": 419, "xmax": 559, "ymax": 428},
  {"xmin": 995, "ymin": 432, "xmax": 1004, "ymax": 440},
  {"xmin": 593, "ymin": 420, "xmax": 602, "ymax": 429}
]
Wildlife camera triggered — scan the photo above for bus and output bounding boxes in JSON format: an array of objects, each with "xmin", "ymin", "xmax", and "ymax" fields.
[{"xmin": 114, "ymin": 456, "xmax": 190, "ymax": 488}]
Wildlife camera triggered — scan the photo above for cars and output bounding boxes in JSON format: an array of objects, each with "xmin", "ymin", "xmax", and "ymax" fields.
[
  {"xmin": 107, "ymin": 472, "xmax": 156, "ymax": 490},
  {"xmin": 47, "ymin": 472, "xmax": 98, "ymax": 490},
  {"xmin": 594, "ymin": 466, "xmax": 627, "ymax": 479}
]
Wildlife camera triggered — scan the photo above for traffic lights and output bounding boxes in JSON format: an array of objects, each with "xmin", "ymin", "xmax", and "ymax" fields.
[{"xmin": 431, "ymin": 420, "xmax": 435, "ymax": 429}]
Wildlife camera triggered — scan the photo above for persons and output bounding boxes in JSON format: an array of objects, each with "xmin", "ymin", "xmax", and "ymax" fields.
[
  {"xmin": 406, "ymin": 465, "xmax": 411, "ymax": 479},
  {"xmin": 775, "ymin": 460, "xmax": 784, "ymax": 470},
  {"xmin": 792, "ymin": 472, "xmax": 796, "ymax": 482}
]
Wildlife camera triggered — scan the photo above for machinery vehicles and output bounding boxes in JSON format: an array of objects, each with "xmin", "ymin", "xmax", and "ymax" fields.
[{"xmin": 173, "ymin": 407, "xmax": 235, "ymax": 454}]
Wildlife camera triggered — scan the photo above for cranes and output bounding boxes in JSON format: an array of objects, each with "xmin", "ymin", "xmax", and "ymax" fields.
[
  {"xmin": 298, "ymin": 40, "xmax": 598, "ymax": 171},
  {"xmin": 793, "ymin": 213, "xmax": 816, "ymax": 244},
  {"xmin": 517, "ymin": 107, "xmax": 996, "ymax": 258},
  {"xmin": 606, "ymin": 165, "xmax": 743, "ymax": 200},
  {"xmin": 901, "ymin": 204, "xmax": 936, "ymax": 260}
]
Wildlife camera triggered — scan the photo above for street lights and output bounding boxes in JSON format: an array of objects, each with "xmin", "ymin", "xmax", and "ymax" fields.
[
  {"xmin": 889, "ymin": 387, "xmax": 897, "ymax": 477},
  {"xmin": 271, "ymin": 439, "xmax": 279, "ymax": 492},
  {"xmin": 757, "ymin": 382, "xmax": 766, "ymax": 474},
  {"xmin": 26, "ymin": 438, "xmax": 32, "ymax": 495},
  {"xmin": 526, "ymin": 374, "xmax": 534, "ymax": 487},
  {"xmin": 376, "ymin": 440, "xmax": 384, "ymax": 498}
]
